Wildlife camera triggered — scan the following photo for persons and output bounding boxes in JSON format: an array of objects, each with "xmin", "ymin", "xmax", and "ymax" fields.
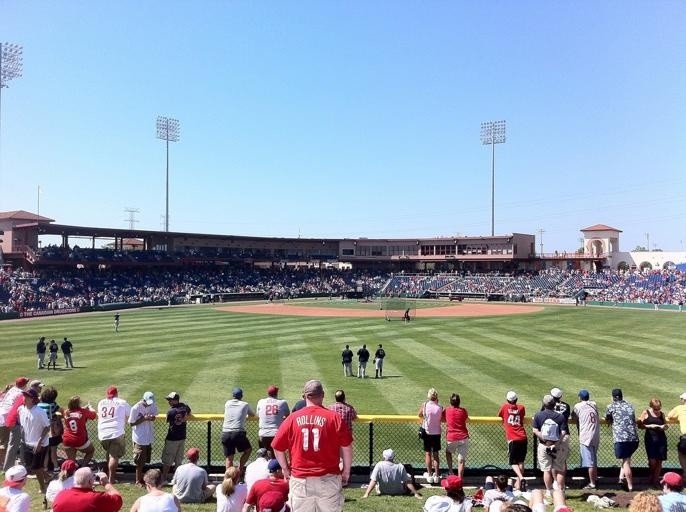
[
  {"xmin": 113, "ymin": 312, "xmax": 120, "ymax": 332},
  {"xmin": 362, "ymin": 389, "xmax": 685, "ymax": 512},
  {"xmin": 35, "ymin": 336, "xmax": 74, "ymax": 371},
  {"xmin": 372, "ymin": 344, "xmax": 385, "ymax": 379},
  {"xmin": 292, "ymin": 390, "xmax": 358, "ymax": 486},
  {"xmin": 1, "ymin": 376, "xmax": 290, "ymax": 512},
  {"xmin": 357, "ymin": 344, "xmax": 369, "ymax": 379},
  {"xmin": 342, "ymin": 345, "xmax": 354, "ymax": 377},
  {"xmin": 405, "ymin": 307, "xmax": 410, "ymax": 323},
  {"xmin": 271, "ymin": 379, "xmax": 353, "ymax": 512},
  {"xmin": 0, "ymin": 243, "xmax": 685, "ymax": 313}
]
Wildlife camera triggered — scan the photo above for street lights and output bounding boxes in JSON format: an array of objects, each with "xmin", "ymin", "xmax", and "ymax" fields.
[
  {"xmin": 0, "ymin": 41, "xmax": 26, "ymax": 88},
  {"xmin": 156, "ymin": 115, "xmax": 181, "ymax": 233},
  {"xmin": 479, "ymin": 117, "xmax": 509, "ymax": 237}
]
